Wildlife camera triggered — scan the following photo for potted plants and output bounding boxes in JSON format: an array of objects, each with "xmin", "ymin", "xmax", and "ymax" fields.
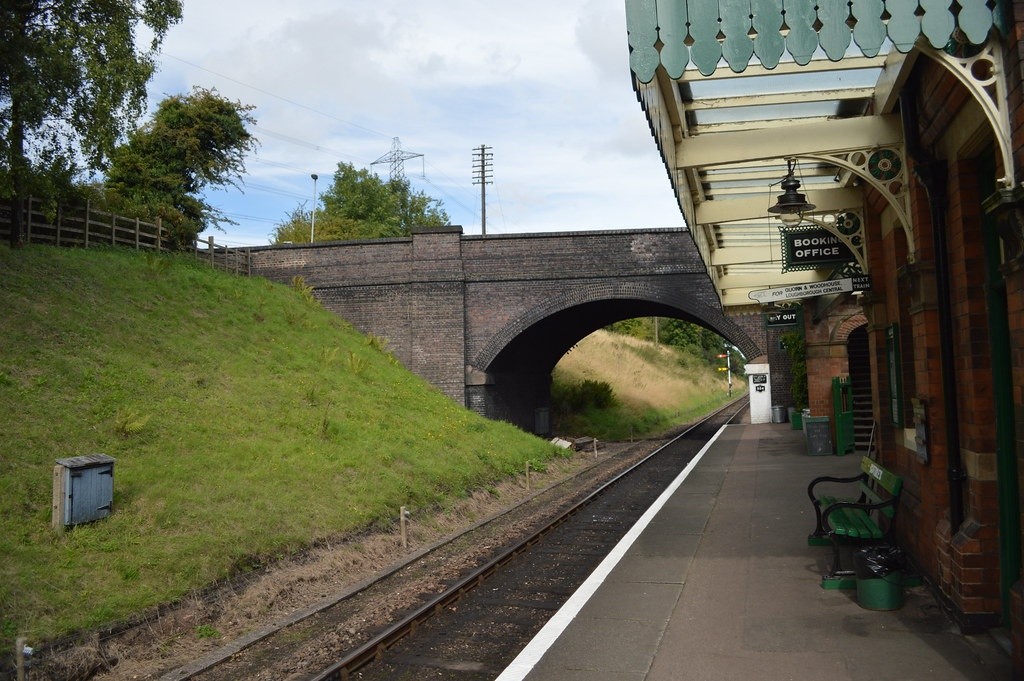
[{"xmin": 778, "ymin": 331, "xmax": 808, "ymax": 430}]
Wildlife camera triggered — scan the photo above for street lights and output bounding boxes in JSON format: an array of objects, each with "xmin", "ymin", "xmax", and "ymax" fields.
[{"xmin": 309, "ymin": 174, "xmax": 318, "ymax": 244}]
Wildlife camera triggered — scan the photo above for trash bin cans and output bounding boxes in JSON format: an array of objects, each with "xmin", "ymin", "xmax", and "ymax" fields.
[
  {"xmin": 772, "ymin": 405, "xmax": 785, "ymax": 424},
  {"xmin": 857, "ymin": 543, "xmax": 911, "ymax": 609}
]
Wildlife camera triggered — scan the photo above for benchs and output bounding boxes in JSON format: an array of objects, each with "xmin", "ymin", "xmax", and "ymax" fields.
[{"xmin": 806, "ymin": 455, "xmax": 923, "ymax": 590}]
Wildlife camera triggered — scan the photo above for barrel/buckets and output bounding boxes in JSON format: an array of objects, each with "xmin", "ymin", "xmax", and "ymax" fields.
[{"xmin": 770, "ymin": 405, "xmax": 785, "ymax": 424}]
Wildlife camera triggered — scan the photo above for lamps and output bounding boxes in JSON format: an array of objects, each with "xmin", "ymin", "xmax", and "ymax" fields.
[{"xmin": 768, "ymin": 155, "xmax": 816, "ymax": 228}]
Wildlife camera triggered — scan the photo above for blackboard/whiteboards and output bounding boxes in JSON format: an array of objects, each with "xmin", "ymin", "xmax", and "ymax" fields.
[{"xmin": 805, "ymin": 415, "xmax": 831, "ymax": 456}]
